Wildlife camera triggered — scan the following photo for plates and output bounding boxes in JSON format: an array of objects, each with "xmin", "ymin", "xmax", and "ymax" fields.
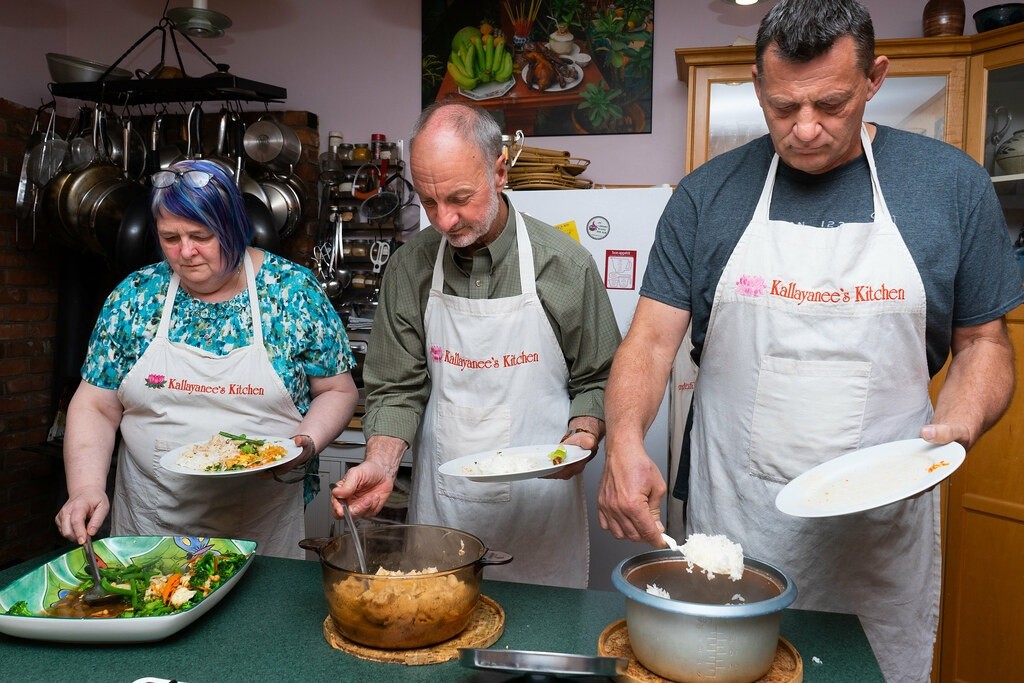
[
  {"xmin": 773, "ymin": 439, "xmax": 967, "ymax": 518},
  {"xmin": 458, "ymin": 74, "xmax": 516, "ymax": 101},
  {"xmin": 522, "ymin": 63, "xmax": 584, "ymax": 92},
  {"xmin": 160, "ymin": 436, "xmax": 303, "ymax": 478},
  {"xmin": 438, "ymin": 444, "xmax": 591, "ymax": 483},
  {"xmin": 0, "ymin": 535, "xmax": 258, "ymax": 642},
  {"xmin": 545, "ymin": 41, "xmax": 581, "ymax": 56}
]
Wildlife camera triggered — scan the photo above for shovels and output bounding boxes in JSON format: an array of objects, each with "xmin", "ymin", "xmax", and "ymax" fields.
[
  {"xmin": 658, "ymin": 533, "xmax": 739, "ymax": 576},
  {"xmin": 82, "ymin": 534, "xmax": 124, "ymax": 604}
]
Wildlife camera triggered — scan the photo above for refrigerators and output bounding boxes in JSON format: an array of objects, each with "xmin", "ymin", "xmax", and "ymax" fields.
[{"xmin": 419, "ymin": 187, "xmax": 672, "ymax": 591}]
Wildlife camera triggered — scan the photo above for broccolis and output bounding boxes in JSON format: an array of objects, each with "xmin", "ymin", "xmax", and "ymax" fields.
[
  {"xmin": 239, "ymin": 442, "xmax": 259, "ymax": 454},
  {"xmin": 5, "ymin": 600, "xmax": 34, "ymax": 616},
  {"xmin": 117, "ymin": 550, "xmax": 246, "ymax": 617}
]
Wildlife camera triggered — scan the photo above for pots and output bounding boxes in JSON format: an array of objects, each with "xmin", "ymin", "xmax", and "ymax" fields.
[
  {"xmin": 613, "ymin": 548, "xmax": 799, "ymax": 683},
  {"xmin": 18, "ymin": 102, "xmax": 310, "ymax": 272},
  {"xmin": 299, "ymin": 524, "xmax": 513, "ymax": 648}
]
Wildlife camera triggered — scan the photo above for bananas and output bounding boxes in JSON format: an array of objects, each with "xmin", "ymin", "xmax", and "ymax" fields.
[{"xmin": 445, "ymin": 34, "xmax": 512, "ymax": 89}]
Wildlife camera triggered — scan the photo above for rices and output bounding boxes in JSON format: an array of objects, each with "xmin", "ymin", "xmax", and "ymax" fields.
[
  {"xmin": 646, "ymin": 583, "xmax": 670, "ymax": 599},
  {"xmin": 682, "ymin": 533, "xmax": 744, "ymax": 580},
  {"xmin": 176, "ymin": 443, "xmax": 240, "ymax": 471}
]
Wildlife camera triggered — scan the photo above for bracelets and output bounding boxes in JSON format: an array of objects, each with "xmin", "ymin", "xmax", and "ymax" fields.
[
  {"xmin": 558, "ymin": 429, "xmax": 600, "ymax": 460},
  {"xmin": 281, "ymin": 434, "xmax": 317, "ymax": 470}
]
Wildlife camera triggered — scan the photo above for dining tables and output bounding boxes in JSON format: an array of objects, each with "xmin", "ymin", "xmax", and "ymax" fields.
[{"xmin": 1, "ymin": 535, "xmax": 889, "ymax": 682}]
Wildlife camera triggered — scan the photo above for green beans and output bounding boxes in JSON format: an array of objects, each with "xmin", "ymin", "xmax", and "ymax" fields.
[
  {"xmin": 75, "ymin": 555, "xmax": 162, "ymax": 610},
  {"xmin": 219, "ymin": 431, "xmax": 264, "ymax": 445}
]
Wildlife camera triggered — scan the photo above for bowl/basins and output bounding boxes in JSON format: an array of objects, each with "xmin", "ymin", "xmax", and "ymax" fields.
[
  {"xmin": 45, "ymin": 52, "xmax": 134, "ymax": 83},
  {"xmin": 576, "ymin": 52, "xmax": 592, "ymax": 67}
]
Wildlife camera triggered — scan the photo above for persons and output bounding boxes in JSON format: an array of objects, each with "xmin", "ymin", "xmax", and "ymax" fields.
[
  {"xmin": 592, "ymin": 0, "xmax": 1024, "ymax": 683},
  {"xmin": 328, "ymin": 100, "xmax": 622, "ymax": 590},
  {"xmin": 52, "ymin": 157, "xmax": 361, "ymax": 549}
]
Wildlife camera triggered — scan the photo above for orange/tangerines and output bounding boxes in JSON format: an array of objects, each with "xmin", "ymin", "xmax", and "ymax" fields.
[{"xmin": 469, "ymin": 23, "xmax": 507, "ymax": 52}]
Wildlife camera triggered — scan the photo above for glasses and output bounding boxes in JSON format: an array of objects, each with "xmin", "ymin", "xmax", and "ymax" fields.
[{"xmin": 149, "ymin": 170, "xmax": 229, "ymax": 196}]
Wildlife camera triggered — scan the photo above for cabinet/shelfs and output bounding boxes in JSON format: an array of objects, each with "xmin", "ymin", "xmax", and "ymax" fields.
[
  {"xmin": 673, "ymin": 34, "xmax": 975, "ymax": 683},
  {"xmin": 318, "ymin": 138, "xmax": 404, "ymax": 331},
  {"xmin": 305, "ymin": 416, "xmax": 412, "ymax": 563},
  {"xmin": 938, "ymin": 20, "xmax": 1024, "ymax": 683}
]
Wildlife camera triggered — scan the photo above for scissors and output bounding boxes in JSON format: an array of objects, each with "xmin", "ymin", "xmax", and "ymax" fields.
[{"xmin": 369, "ymin": 241, "xmax": 391, "ymax": 273}]
